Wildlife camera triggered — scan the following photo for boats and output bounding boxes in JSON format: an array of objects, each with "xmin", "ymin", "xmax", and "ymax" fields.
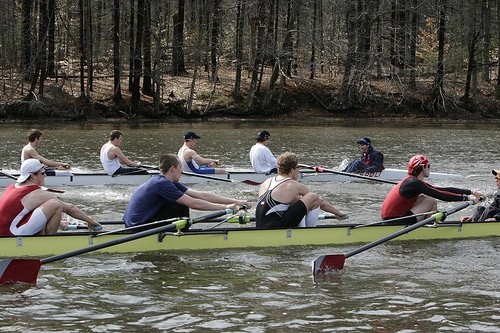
[
  {"xmin": 0, "ymin": 159, "xmax": 466, "ymax": 186},
  {"xmin": 0, "ymin": 221, "xmax": 500, "ymax": 259}
]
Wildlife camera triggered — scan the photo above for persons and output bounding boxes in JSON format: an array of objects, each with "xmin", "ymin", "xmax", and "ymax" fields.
[
  {"xmin": 381, "ymin": 154, "xmax": 484, "ymax": 224},
  {"xmin": 255, "ymin": 151, "xmax": 347, "ymax": 229},
  {"xmin": 249, "ymin": 130, "xmax": 278, "ymax": 175},
  {"xmin": 100, "ymin": 130, "xmax": 148, "ymax": 177},
  {"xmin": 20, "ymin": 129, "xmax": 70, "ymax": 170},
  {"xmin": 0, "ymin": 158, "xmax": 102, "ymax": 237},
  {"xmin": 177, "ymin": 132, "xmax": 229, "ymax": 175},
  {"xmin": 342, "ymin": 137, "xmax": 385, "ymax": 173},
  {"xmin": 122, "ymin": 154, "xmax": 252, "ymax": 232}
]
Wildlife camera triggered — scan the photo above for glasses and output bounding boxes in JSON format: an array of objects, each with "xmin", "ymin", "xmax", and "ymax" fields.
[
  {"xmin": 28, "ymin": 172, "xmax": 44, "ymax": 175},
  {"xmin": 360, "ymin": 139, "xmax": 370, "ymax": 144},
  {"xmin": 419, "ymin": 163, "xmax": 430, "ymax": 169}
]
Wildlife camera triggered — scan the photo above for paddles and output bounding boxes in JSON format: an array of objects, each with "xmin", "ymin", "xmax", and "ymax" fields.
[
  {"xmin": 298, "ymin": 163, "xmax": 399, "ymax": 185},
  {"xmin": 140, "ymin": 165, "xmax": 261, "ymax": 186},
  {"xmin": 99, "ymin": 215, "xmax": 349, "ymax": 224},
  {"xmin": 312, "ymin": 196, "xmax": 485, "ymax": 283},
  {"xmin": 199, "ymin": 162, "xmax": 219, "ymax": 167},
  {"xmin": 68, "ymin": 225, "xmax": 103, "ymax": 231},
  {"xmin": 0, "ymin": 205, "xmax": 246, "ymax": 286},
  {"xmin": 0, "ymin": 172, "xmax": 66, "ymax": 193}
]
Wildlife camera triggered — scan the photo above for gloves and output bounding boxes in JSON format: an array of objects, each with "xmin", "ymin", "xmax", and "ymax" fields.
[{"xmin": 86, "ymin": 217, "xmax": 102, "ymax": 231}]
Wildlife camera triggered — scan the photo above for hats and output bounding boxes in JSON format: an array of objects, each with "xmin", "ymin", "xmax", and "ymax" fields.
[
  {"xmin": 492, "ymin": 170, "xmax": 500, "ymax": 178},
  {"xmin": 255, "ymin": 130, "xmax": 271, "ymax": 140},
  {"xmin": 185, "ymin": 132, "xmax": 201, "ymax": 140},
  {"xmin": 407, "ymin": 155, "xmax": 429, "ymax": 174},
  {"xmin": 17, "ymin": 158, "xmax": 44, "ymax": 183},
  {"xmin": 357, "ymin": 137, "xmax": 371, "ymax": 145}
]
